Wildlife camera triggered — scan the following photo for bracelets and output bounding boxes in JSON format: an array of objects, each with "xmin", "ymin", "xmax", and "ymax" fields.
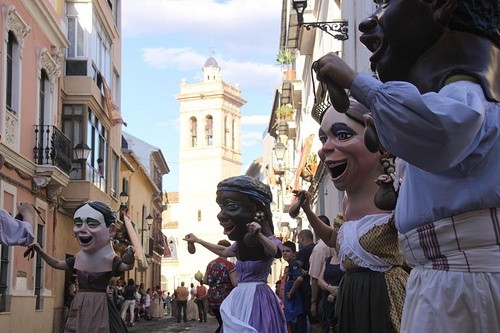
[{"xmin": 312, "ymin": 298, "xmax": 316, "ymax": 303}]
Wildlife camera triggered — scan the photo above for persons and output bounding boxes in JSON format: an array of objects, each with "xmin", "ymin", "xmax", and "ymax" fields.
[
  {"xmin": 172, "ymin": 281, "xmax": 207, "ymax": 323},
  {"xmin": 203, "ymin": 240, "xmax": 237, "ymax": 333},
  {"xmin": 183, "ymin": 175, "xmax": 288, "ymax": 333},
  {"xmin": 308, "ymin": 213, "xmax": 345, "ymax": 333},
  {"xmin": 0, "ymin": 202, "xmax": 35, "ymax": 247},
  {"xmin": 135, "ymin": 283, "xmax": 162, "ymax": 320},
  {"xmin": 276, "ymin": 230, "xmax": 324, "ymax": 333},
  {"xmin": 313, "ymin": 0, "xmax": 500, "ymax": 333},
  {"xmin": 28, "ymin": 201, "xmax": 136, "ymax": 333},
  {"xmin": 161, "ymin": 290, "xmax": 172, "ymax": 315},
  {"xmin": 69, "ymin": 273, "xmax": 78, "ymax": 297},
  {"xmin": 292, "ymin": 88, "xmax": 410, "ymax": 333},
  {"xmin": 108, "ymin": 279, "xmax": 136, "ymax": 322}
]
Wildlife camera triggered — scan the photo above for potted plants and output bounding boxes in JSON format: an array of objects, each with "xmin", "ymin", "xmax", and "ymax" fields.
[{"xmin": 275, "ymin": 105, "xmax": 294, "ymax": 120}]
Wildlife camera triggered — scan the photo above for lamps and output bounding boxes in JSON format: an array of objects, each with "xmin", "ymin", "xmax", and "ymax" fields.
[
  {"xmin": 292, "ymin": 0, "xmax": 349, "ymax": 41},
  {"xmin": 67, "ymin": 140, "xmax": 91, "ymax": 174},
  {"xmin": 138, "ymin": 214, "xmax": 154, "ymax": 234},
  {"xmin": 276, "ymin": 50, "xmax": 299, "ymax": 80},
  {"xmin": 272, "ymin": 138, "xmax": 297, "ymax": 175},
  {"xmin": 120, "ymin": 191, "xmax": 129, "ymax": 209}
]
